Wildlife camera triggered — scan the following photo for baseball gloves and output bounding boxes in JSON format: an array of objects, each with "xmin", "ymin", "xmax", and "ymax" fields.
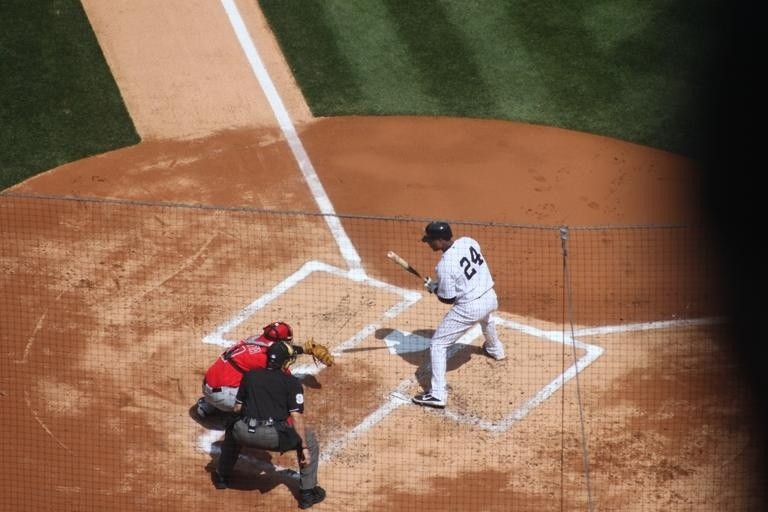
[{"xmin": 303, "ymin": 337, "xmax": 336, "ymax": 368}]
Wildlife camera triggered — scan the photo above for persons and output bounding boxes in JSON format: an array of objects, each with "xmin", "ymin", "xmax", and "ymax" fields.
[
  {"xmin": 195, "ymin": 320, "xmax": 294, "ymax": 416},
  {"xmin": 410, "ymin": 219, "xmax": 506, "ymax": 408},
  {"xmin": 212, "ymin": 339, "xmax": 328, "ymax": 509}
]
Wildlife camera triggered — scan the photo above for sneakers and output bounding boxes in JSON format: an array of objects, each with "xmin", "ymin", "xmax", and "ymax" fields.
[
  {"xmin": 483, "ymin": 340, "xmax": 505, "ymax": 360},
  {"xmin": 300, "ymin": 486, "xmax": 326, "ymax": 508},
  {"xmin": 214, "ymin": 470, "xmax": 229, "ymax": 489},
  {"xmin": 197, "ymin": 398, "xmax": 213, "ymax": 418},
  {"xmin": 412, "ymin": 393, "xmax": 446, "ymax": 406}
]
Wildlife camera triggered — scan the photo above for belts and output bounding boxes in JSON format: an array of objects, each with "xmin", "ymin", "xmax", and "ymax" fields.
[{"xmin": 241, "ymin": 415, "xmax": 273, "ymax": 426}]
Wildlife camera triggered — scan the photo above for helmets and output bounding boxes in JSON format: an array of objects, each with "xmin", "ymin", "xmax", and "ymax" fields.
[
  {"xmin": 264, "ymin": 321, "xmax": 292, "ymax": 344},
  {"xmin": 266, "ymin": 341, "xmax": 298, "ymax": 370},
  {"xmin": 422, "ymin": 220, "xmax": 451, "ymax": 242}
]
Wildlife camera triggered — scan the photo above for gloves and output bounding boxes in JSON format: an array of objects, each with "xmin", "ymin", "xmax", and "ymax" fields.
[{"xmin": 424, "ymin": 276, "xmax": 438, "ymax": 294}]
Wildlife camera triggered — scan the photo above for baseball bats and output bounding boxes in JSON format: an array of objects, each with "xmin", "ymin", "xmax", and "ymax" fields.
[{"xmin": 387, "ymin": 251, "xmax": 427, "ymax": 282}]
